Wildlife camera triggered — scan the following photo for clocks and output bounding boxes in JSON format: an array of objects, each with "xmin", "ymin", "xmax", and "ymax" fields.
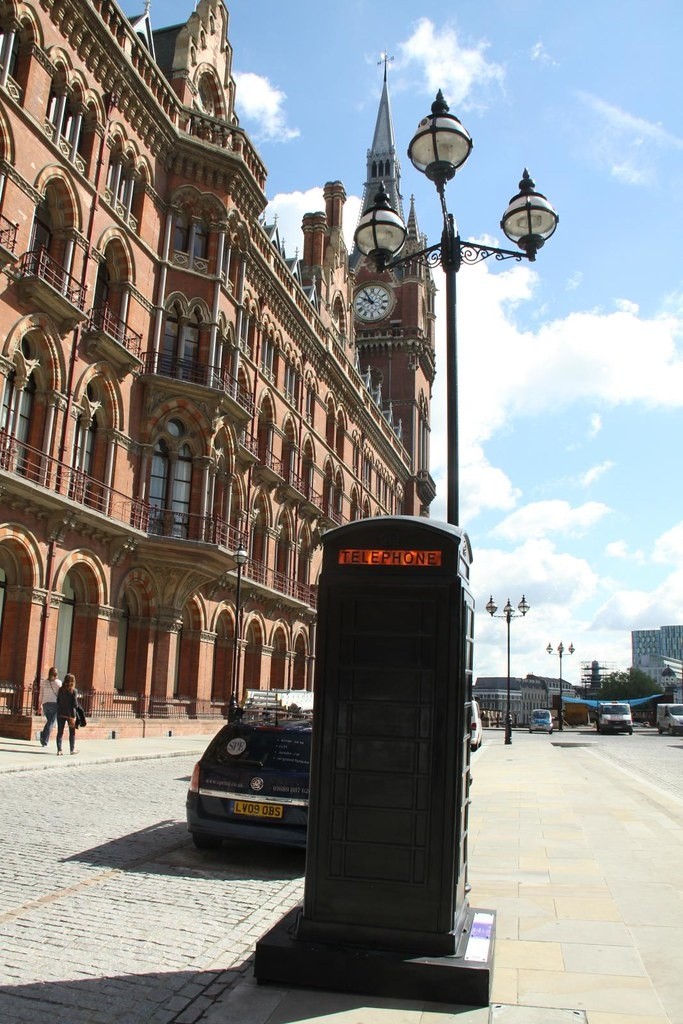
[{"xmin": 352, "ymin": 284, "xmax": 395, "ymax": 323}]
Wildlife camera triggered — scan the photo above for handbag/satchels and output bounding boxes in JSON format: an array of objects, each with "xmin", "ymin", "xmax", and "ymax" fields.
[{"xmin": 70, "ymin": 718, "xmax": 76, "ymax": 728}]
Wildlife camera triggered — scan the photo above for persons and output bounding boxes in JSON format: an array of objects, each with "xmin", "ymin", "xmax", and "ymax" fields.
[
  {"xmin": 56, "ymin": 673, "xmax": 80, "ymax": 756},
  {"xmin": 39, "ymin": 667, "xmax": 62, "ymax": 747}
]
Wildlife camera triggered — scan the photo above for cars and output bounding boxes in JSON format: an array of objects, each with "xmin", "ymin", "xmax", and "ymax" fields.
[
  {"xmin": 470, "ymin": 700, "xmax": 486, "ymax": 753},
  {"xmin": 529, "ymin": 708, "xmax": 555, "ymax": 734},
  {"xmin": 185, "ymin": 695, "xmax": 312, "ymax": 856}
]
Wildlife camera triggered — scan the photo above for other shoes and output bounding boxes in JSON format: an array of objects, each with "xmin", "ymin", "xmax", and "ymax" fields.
[
  {"xmin": 57, "ymin": 751, "xmax": 63, "ymax": 755},
  {"xmin": 70, "ymin": 750, "xmax": 79, "ymax": 755}
]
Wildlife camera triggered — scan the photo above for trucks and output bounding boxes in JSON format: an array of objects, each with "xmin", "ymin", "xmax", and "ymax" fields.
[{"xmin": 594, "ymin": 700, "xmax": 633, "ymax": 735}]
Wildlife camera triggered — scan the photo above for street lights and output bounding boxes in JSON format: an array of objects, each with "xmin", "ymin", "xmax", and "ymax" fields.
[
  {"xmin": 547, "ymin": 642, "xmax": 576, "ymax": 730},
  {"xmin": 352, "ymin": 86, "xmax": 560, "ymax": 528},
  {"xmin": 486, "ymin": 593, "xmax": 531, "ymax": 745},
  {"xmin": 226, "ymin": 538, "xmax": 248, "ymax": 723}
]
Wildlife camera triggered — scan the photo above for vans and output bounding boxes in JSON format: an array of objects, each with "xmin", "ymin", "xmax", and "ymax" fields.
[{"xmin": 656, "ymin": 704, "xmax": 683, "ymax": 735}]
